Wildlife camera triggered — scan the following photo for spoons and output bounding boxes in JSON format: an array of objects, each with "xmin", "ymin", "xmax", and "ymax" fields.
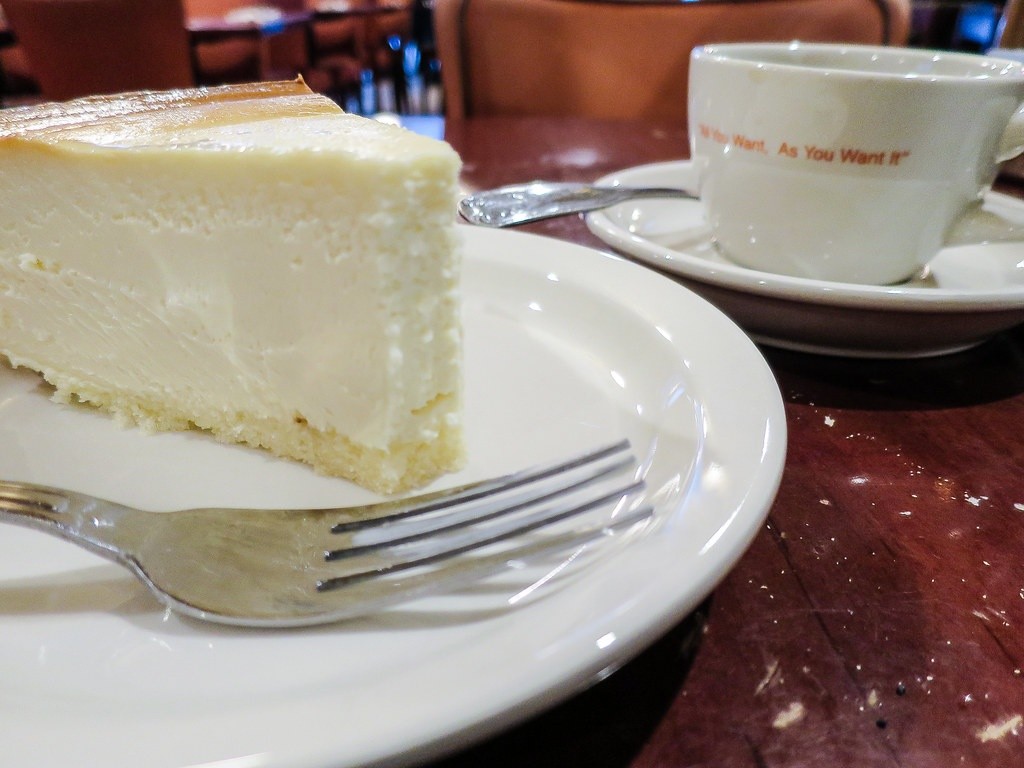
[{"xmin": 454, "ymin": 182, "xmax": 698, "ymax": 229}]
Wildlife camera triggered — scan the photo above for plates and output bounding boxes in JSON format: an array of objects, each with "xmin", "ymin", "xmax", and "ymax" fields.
[
  {"xmin": 580, "ymin": 158, "xmax": 1024, "ymax": 360},
  {"xmin": 1, "ymin": 224, "xmax": 790, "ymax": 768}
]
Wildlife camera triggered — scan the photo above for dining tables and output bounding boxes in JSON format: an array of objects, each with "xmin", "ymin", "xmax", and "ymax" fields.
[{"xmin": 398, "ymin": 116, "xmax": 1024, "ymax": 768}]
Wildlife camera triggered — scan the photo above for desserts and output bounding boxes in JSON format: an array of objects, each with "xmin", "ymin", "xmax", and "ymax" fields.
[{"xmin": 0, "ymin": 73, "xmax": 463, "ymax": 495}]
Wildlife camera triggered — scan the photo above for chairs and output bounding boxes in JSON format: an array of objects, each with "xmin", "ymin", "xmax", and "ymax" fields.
[
  {"xmin": 432, "ymin": 0, "xmax": 911, "ymax": 126},
  {"xmin": 3, "ymin": 0, "xmax": 192, "ymax": 101}
]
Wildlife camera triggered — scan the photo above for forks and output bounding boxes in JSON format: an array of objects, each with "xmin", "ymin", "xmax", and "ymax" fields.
[{"xmin": 0, "ymin": 438, "xmax": 658, "ymax": 630}]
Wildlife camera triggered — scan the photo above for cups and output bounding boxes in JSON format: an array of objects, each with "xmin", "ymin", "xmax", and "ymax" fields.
[{"xmin": 684, "ymin": 42, "xmax": 1022, "ymax": 287}]
